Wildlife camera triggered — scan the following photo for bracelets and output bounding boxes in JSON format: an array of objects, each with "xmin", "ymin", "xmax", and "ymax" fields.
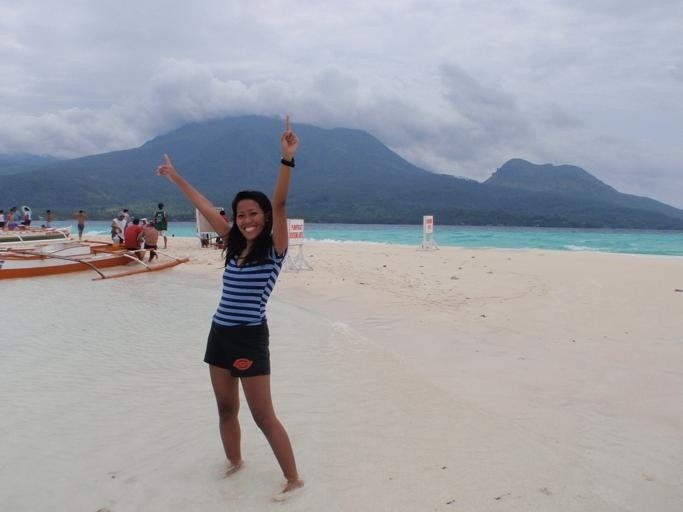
[{"xmin": 280, "ymin": 157, "xmax": 295, "ymax": 167}]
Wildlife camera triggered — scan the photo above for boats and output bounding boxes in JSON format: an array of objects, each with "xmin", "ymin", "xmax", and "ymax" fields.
[
  {"xmin": 0, "ymin": 225, "xmax": 75, "ymax": 241},
  {"xmin": 0, "ymin": 238, "xmax": 191, "ymax": 281}
]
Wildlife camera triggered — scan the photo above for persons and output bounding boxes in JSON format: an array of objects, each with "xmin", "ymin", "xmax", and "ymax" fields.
[
  {"xmin": 0, "ymin": 203, "xmax": 229, "ymax": 262},
  {"xmin": 156, "ymin": 114, "xmax": 302, "ymax": 501}
]
